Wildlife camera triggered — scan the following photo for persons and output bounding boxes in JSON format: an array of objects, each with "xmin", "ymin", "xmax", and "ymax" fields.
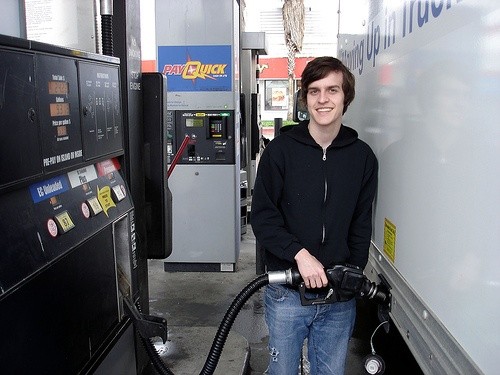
[{"xmin": 250, "ymin": 57, "xmax": 378, "ymax": 375}]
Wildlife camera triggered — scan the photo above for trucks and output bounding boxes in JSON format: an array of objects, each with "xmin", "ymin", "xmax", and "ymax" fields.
[{"xmin": 293, "ymin": 0, "xmax": 500, "ymax": 375}]
